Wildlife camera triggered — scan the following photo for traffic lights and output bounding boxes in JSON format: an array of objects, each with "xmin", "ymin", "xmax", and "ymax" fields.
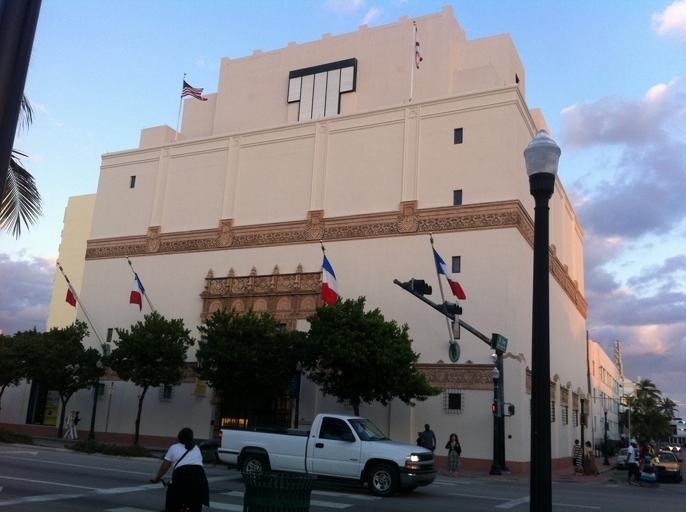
[
  {"xmin": 507, "ymin": 404, "xmax": 516, "ymax": 416},
  {"xmin": 491, "ymin": 402, "xmax": 498, "ymax": 416},
  {"xmin": 408, "ymin": 277, "xmax": 434, "ymax": 299},
  {"xmin": 443, "ymin": 302, "xmax": 463, "ymax": 316}
]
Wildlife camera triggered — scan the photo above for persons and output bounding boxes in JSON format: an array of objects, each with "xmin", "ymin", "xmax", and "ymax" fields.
[
  {"xmin": 417, "ymin": 423, "xmax": 437, "ymax": 450},
  {"xmin": 61, "ymin": 410, "xmax": 81, "ymax": 440},
  {"xmin": 151, "ymin": 427, "xmax": 210, "ymax": 512},
  {"xmin": 445, "ymin": 431, "xmax": 461, "ymax": 475},
  {"xmin": 572, "ymin": 440, "xmax": 601, "ymax": 477},
  {"xmin": 625, "ymin": 440, "xmax": 643, "ymax": 486}
]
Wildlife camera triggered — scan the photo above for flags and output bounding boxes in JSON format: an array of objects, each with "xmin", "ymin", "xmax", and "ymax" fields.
[
  {"xmin": 181, "ymin": 80, "xmax": 208, "ymax": 101},
  {"xmin": 129, "ymin": 272, "xmax": 145, "ymax": 312},
  {"xmin": 65, "ymin": 282, "xmax": 79, "ymax": 307},
  {"xmin": 433, "ymin": 246, "xmax": 466, "ymax": 301},
  {"xmin": 416, "ymin": 40, "xmax": 423, "ymax": 69},
  {"xmin": 321, "ymin": 255, "xmax": 337, "ymax": 306}
]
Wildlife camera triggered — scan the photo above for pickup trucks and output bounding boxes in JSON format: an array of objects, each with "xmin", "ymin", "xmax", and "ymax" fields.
[{"xmin": 216, "ymin": 410, "xmax": 441, "ymax": 499}]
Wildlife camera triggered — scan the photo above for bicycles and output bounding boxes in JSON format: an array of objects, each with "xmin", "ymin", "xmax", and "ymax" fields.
[{"xmin": 149, "ymin": 477, "xmax": 192, "ymax": 512}]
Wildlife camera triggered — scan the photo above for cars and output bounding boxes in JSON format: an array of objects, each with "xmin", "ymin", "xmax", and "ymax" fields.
[{"xmin": 613, "ymin": 441, "xmax": 683, "ymax": 485}]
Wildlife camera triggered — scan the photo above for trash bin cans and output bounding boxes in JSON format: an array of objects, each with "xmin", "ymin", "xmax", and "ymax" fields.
[{"xmin": 241, "ymin": 471, "xmax": 318, "ymax": 512}]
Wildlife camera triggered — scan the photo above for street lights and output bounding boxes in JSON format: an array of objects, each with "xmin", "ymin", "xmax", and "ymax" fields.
[
  {"xmin": 602, "ymin": 406, "xmax": 611, "ymax": 465},
  {"xmin": 519, "ymin": 122, "xmax": 569, "ymax": 512},
  {"xmin": 490, "ymin": 367, "xmax": 504, "ymax": 475},
  {"xmin": 579, "ymin": 391, "xmax": 587, "ymax": 465}
]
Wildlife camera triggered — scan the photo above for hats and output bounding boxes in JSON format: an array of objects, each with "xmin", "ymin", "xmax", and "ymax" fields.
[{"xmin": 630, "ymin": 439, "xmax": 638, "ymax": 444}]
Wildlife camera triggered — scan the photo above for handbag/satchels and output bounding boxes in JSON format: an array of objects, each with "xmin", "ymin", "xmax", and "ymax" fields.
[
  {"xmin": 159, "ymin": 466, "xmax": 174, "ymax": 485},
  {"xmin": 572, "ymin": 457, "xmax": 577, "ymax": 466}
]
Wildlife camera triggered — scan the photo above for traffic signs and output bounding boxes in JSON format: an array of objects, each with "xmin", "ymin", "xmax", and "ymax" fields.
[{"xmin": 494, "ymin": 334, "xmax": 509, "ymax": 353}]
